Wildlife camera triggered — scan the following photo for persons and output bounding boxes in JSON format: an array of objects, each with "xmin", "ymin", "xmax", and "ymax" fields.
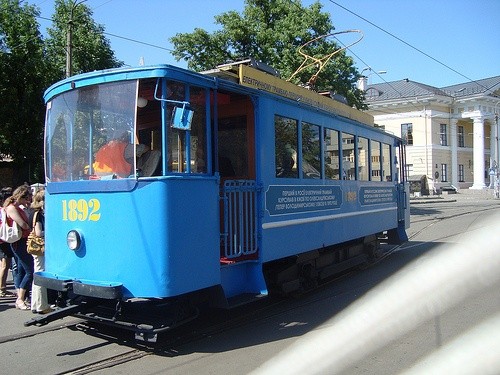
[
  {"xmin": 91, "ymin": 129, "xmax": 149, "ymax": 178},
  {"xmin": 1, "ymin": 183, "xmax": 33, "ymax": 310},
  {"xmin": 0, "ymin": 187, "xmax": 14, "ymax": 296},
  {"xmin": 27, "ymin": 181, "xmax": 57, "ymax": 314}
]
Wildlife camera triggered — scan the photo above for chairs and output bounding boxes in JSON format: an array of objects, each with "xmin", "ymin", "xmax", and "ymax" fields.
[{"xmin": 132, "ymin": 150, "xmax": 161, "ymax": 177}]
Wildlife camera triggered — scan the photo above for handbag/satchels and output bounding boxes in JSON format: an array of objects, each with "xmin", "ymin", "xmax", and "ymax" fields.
[
  {"xmin": 27, "ymin": 210, "xmax": 45, "ymax": 256},
  {"xmin": 0, "ymin": 213, "xmax": 22, "ymax": 244}
]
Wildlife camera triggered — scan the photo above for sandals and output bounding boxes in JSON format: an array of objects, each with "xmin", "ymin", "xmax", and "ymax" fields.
[{"xmin": 13, "ymin": 295, "xmax": 32, "ymax": 312}]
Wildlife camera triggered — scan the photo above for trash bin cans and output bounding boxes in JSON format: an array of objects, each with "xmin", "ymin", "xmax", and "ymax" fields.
[{"xmin": 408, "ymin": 175, "xmax": 428, "ymax": 196}]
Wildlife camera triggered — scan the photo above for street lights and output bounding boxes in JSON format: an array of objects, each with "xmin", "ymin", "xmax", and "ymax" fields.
[{"xmin": 358, "ymin": 66, "xmax": 387, "ymax": 87}]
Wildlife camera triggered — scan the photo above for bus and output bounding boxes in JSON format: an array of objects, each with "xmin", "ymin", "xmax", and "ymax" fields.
[{"xmin": 24, "ymin": 59, "xmax": 410, "ymax": 342}]
